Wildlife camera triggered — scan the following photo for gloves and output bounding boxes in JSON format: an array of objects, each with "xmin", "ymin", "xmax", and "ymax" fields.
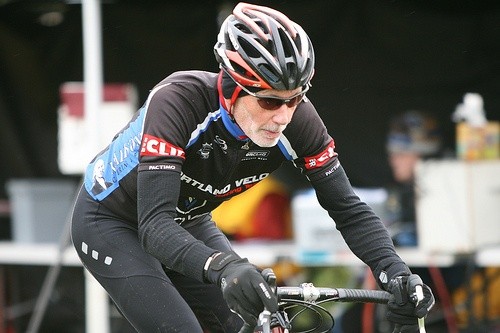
[
  {"xmin": 373, "ymin": 255, "xmax": 435, "ymax": 330},
  {"xmin": 207, "ymin": 251, "xmax": 278, "ymax": 326}
]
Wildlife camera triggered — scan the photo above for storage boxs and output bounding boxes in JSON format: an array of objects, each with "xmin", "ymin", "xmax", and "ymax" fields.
[
  {"xmin": 58, "ymin": 82, "xmax": 137, "ymax": 174},
  {"xmin": 7, "ymin": 178, "xmax": 77, "ymax": 244},
  {"xmin": 291, "ymin": 187, "xmax": 387, "ymax": 267},
  {"xmin": 413, "ymin": 159, "xmax": 500, "ymax": 253}
]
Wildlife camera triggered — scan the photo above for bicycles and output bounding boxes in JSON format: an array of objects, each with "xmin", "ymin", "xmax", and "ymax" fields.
[{"xmin": 190, "ymin": 268, "xmax": 424, "ymax": 332}]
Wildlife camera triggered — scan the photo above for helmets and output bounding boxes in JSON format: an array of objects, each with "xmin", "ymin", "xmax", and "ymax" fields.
[{"xmin": 213, "ymin": 2, "xmax": 315, "ymax": 90}]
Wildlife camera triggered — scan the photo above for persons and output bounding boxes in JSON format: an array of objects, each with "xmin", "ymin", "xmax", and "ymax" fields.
[
  {"xmin": 69, "ymin": 1, "xmax": 438, "ymax": 333},
  {"xmin": 91, "ymin": 158, "xmax": 113, "ymax": 195},
  {"xmin": 378, "ymin": 110, "xmax": 475, "ymax": 333}
]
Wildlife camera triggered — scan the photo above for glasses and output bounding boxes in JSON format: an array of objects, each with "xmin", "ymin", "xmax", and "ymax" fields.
[{"xmin": 220, "ymin": 66, "xmax": 309, "ymax": 109}]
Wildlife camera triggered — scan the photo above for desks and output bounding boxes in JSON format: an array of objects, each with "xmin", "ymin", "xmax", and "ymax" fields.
[{"xmin": 0, "ymin": 241, "xmax": 500, "ymax": 333}]
